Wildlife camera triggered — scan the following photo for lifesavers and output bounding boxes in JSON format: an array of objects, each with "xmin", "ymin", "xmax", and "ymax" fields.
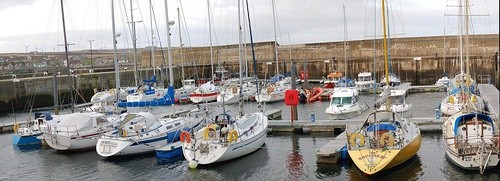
[
  {"xmin": 449, "ymin": 95, "xmax": 453, "ymax": 103},
  {"xmin": 351, "ymin": 133, "xmax": 365, "ymax": 147},
  {"xmin": 471, "ymin": 95, "xmax": 477, "ymax": 103},
  {"xmin": 228, "ymin": 130, "xmax": 238, "ymax": 143},
  {"xmin": 14, "ymin": 122, "xmax": 19, "ymax": 131},
  {"xmin": 180, "ymin": 131, "xmax": 190, "ymax": 143},
  {"xmin": 380, "ymin": 132, "xmax": 394, "ymax": 146},
  {"xmin": 204, "ymin": 127, "xmax": 217, "ymax": 140}
]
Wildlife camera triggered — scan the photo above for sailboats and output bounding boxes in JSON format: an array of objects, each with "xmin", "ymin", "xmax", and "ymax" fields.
[{"xmin": 12, "ymin": 0, "xmax": 500, "ymax": 176}]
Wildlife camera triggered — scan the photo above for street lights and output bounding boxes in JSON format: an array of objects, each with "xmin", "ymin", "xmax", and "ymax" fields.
[{"xmin": 88, "ymin": 39, "xmax": 94, "ymax": 68}]
